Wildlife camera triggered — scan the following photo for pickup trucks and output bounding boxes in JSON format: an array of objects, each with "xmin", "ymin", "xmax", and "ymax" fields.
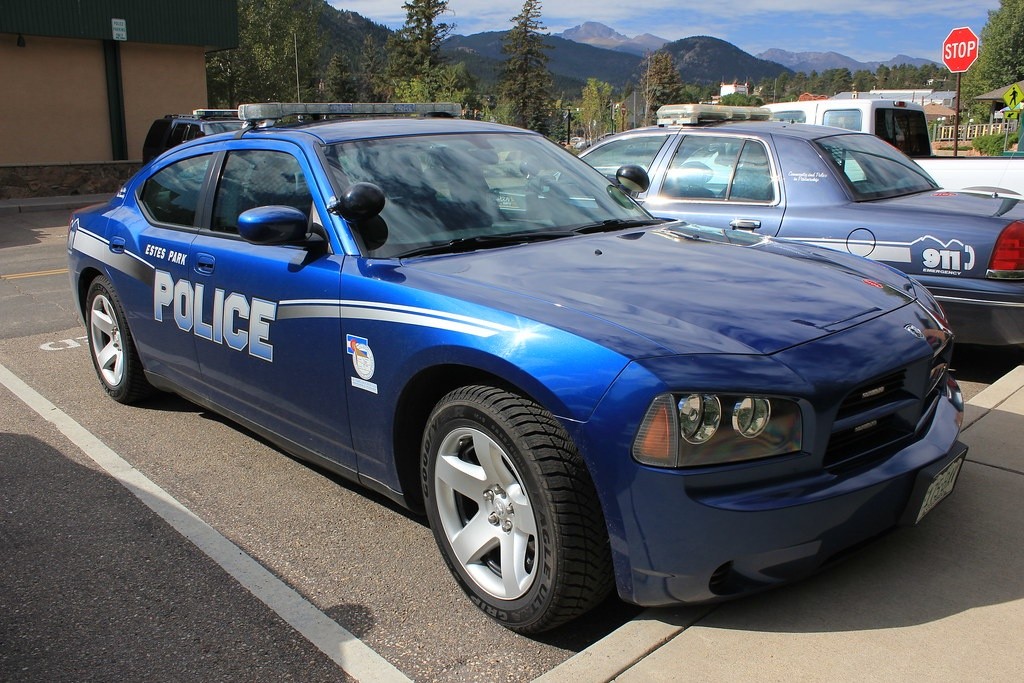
[{"xmin": 677, "ymin": 95, "xmax": 1024, "ymax": 202}]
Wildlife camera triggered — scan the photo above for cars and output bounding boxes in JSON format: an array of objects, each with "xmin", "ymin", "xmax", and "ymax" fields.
[
  {"xmin": 63, "ymin": 99, "xmax": 972, "ymax": 636},
  {"xmin": 484, "ymin": 104, "xmax": 1024, "ymax": 355}
]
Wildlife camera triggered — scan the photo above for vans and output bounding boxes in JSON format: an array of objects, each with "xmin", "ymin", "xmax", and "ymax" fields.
[{"xmin": 142, "ymin": 109, "xmax": 353, "ymax": 220}]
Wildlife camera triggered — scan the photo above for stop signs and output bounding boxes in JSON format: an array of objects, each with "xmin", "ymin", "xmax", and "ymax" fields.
[{"xmin": 942, "ymin": 25, "xmax": 980, "ymax": 74}]
